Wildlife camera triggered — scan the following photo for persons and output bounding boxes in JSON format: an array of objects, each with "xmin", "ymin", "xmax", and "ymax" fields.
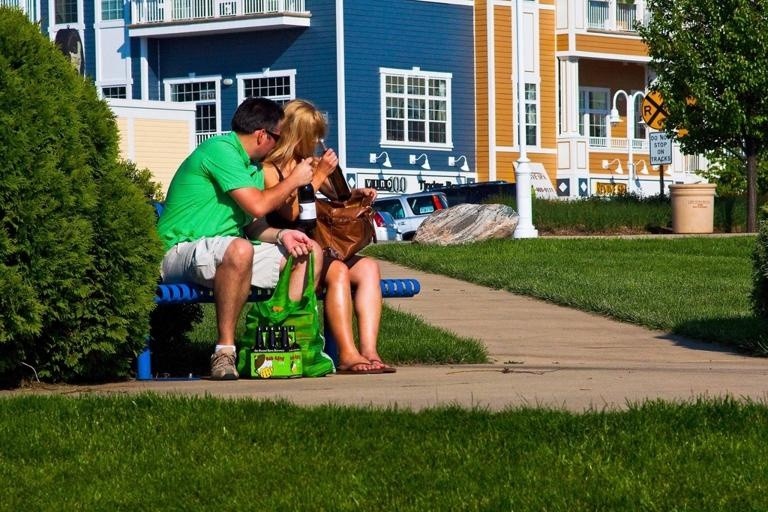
[
  {"xmin": 263, "ymin": 97, "xmax": 396, "ymax": 375},
  {"xmin": 155, "ymin": 96, "xmax": 324, "ymax": 379}
]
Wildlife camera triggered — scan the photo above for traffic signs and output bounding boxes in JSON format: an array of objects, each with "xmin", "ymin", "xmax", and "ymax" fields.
[{"xmin": 648, "ymin": 132, "xmax": 672, "ymax": 166}]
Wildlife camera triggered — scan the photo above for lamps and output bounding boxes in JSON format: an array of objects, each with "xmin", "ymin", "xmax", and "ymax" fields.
[
  {"xmin": 602, "ymin": 158, "xmax": 650, "ymax": 175},
  {"xmin": 369, "ymin": 151, "xmax": 471, "ymax": 172}
]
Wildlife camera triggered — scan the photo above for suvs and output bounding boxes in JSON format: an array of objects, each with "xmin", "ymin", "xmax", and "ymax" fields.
[{"xmin": 370, "ymin": 179, "xmax": 517, "ymax": 243}]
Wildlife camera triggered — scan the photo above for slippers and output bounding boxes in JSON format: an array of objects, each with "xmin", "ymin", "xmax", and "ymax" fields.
[{"xmin": 337, "ymin": 360, "xmax": 396, "ymax": 374}]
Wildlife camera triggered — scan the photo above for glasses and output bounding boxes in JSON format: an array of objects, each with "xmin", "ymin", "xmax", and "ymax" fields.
[{"xmin": 265, "ymin": 129, "xmax": 281, "ymax": 142}]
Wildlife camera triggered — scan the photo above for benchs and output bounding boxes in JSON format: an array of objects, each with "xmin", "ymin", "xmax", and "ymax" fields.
[{"xmin": 137, "ymin": 202, "xmax": 421, "ymax": 380}]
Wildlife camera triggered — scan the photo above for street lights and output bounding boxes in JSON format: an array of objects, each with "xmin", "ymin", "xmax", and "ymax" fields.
[{"xmin": 608, "ymin": 89, "xmax": 647, "ymax": 199}]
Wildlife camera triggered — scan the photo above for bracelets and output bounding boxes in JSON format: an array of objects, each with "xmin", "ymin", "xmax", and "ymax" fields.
[{"xmin": 276, "ymin": 228, "xmax": 291, "ymax": 246}]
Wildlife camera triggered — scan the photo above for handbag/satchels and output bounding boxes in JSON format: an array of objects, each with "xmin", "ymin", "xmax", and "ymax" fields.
[
  {"xmin": 314, "ymin": 189, "xmax": 376, "ymax": 261},
  {"xmin": 237, "ymin": 249, "xmax": 336, "ymax": 378}
]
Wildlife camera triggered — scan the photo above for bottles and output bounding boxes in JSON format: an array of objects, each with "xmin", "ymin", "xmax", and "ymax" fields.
[
  {"xmin": 253, "ymin": 325, "xmax": 300, "ymax": 352},
  {"xmin": 319, "ymin": 138, "xmax": 353, "ymax": 202},
  {"xmin": 297, "ymin": 183, "xmax": 318, "ymax": 229}
]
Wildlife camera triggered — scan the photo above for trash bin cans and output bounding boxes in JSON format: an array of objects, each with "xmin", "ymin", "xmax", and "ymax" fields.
[{"xmin": 668, "ymin": 183, "xmax": 718, "ymax": 233}]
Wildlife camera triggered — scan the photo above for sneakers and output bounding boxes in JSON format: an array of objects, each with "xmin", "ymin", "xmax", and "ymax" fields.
[{"xmin": 210, "ymin": 347, "xmax": 239, "ymax": 380}]
